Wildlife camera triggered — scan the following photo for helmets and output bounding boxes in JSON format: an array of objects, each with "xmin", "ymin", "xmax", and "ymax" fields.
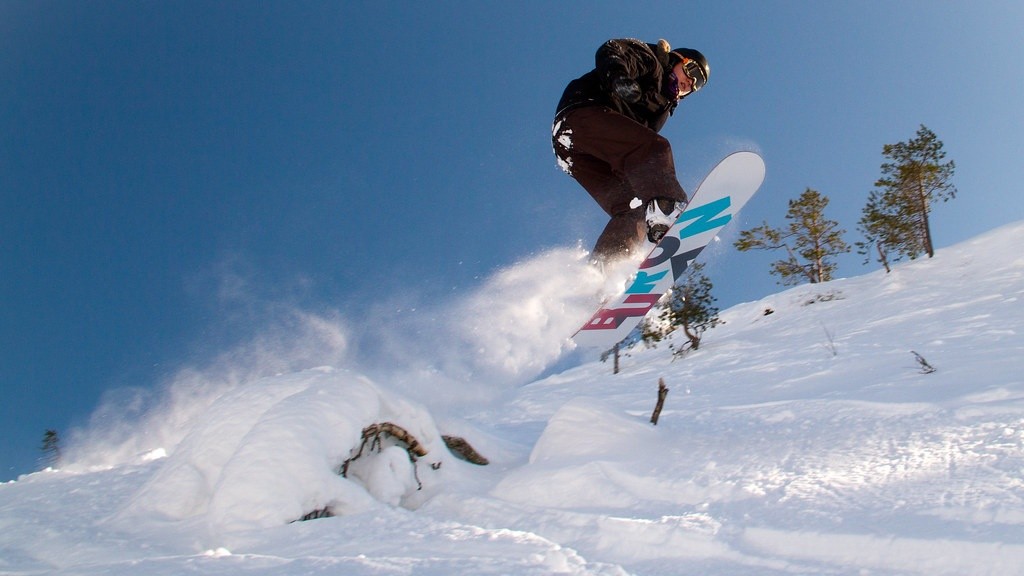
[{"xmin": 667, "ymin": 47, "xmax": 709, "ymax": 84}]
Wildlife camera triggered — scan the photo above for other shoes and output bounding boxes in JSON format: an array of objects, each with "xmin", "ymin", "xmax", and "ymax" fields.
[{"xmin": 644, "ymin": 199, "xmax": 684, "ymax": 243}]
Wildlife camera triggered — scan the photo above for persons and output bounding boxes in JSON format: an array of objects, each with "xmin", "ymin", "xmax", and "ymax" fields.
[{"xmin": 551, "ymin": 38, "xmax": 710, "ymax": 269}]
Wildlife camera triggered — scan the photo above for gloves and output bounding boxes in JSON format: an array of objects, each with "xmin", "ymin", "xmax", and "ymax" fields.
[{"xmin": 614, "ymin": 76, "xmax": 641, "ymax": 103}]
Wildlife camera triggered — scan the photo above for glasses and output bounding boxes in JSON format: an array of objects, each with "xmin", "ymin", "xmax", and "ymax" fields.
[{"xmin": 682, "ymin": 57, "xmax": 706, "ymax": 91}]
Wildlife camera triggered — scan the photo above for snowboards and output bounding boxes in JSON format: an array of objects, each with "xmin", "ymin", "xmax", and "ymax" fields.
[{"xmin": 571, "ymin": 151, "xmax": 766, "ymax": 348}]
